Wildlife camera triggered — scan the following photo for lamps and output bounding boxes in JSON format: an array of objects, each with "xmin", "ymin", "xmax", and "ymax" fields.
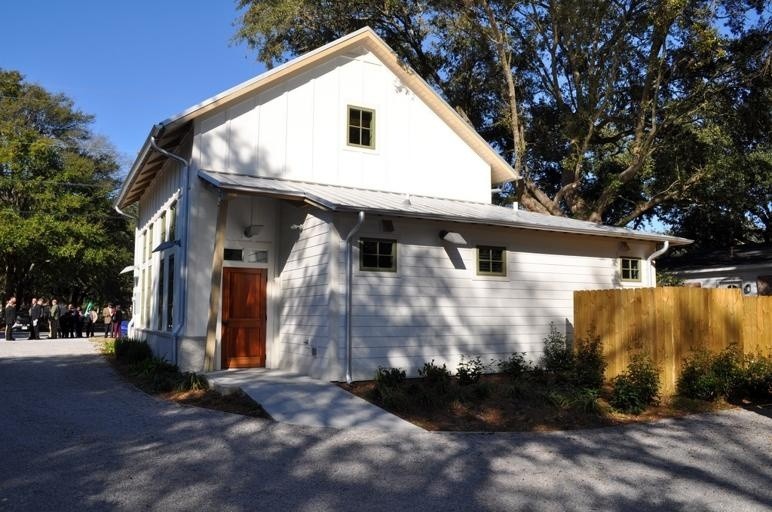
[
  {"xmin": 440, "ymin": 229, "xmax": 467, "ymax": 247},
  {"xmin": 119, "ymin": 265, "xmax": 138, "ymax": 274},
  {"xmin": 152, "ymin": 238, "xmax": 182, "ymax": 253},
  {"xmin": 244, "ymin": 225, "xmax": 264, "ymax": 238}
]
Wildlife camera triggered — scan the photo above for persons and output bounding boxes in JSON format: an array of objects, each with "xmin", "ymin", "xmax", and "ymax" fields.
[
  {"xmin": 3, "ymin": 295, "xmax": 132, "ymax": 341},
  {"xmin": 756, "ymin": 275, "xmax": 772, "ymax": 298}
]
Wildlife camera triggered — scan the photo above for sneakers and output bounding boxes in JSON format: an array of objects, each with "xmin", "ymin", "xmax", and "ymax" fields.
[
  {"xmin": 4, "ymin": 332, "xmax": 95, "ymax": 340},
  {"xmin": 105, "ymin": 333, "xmax": 121, "ymax": 339}
]
